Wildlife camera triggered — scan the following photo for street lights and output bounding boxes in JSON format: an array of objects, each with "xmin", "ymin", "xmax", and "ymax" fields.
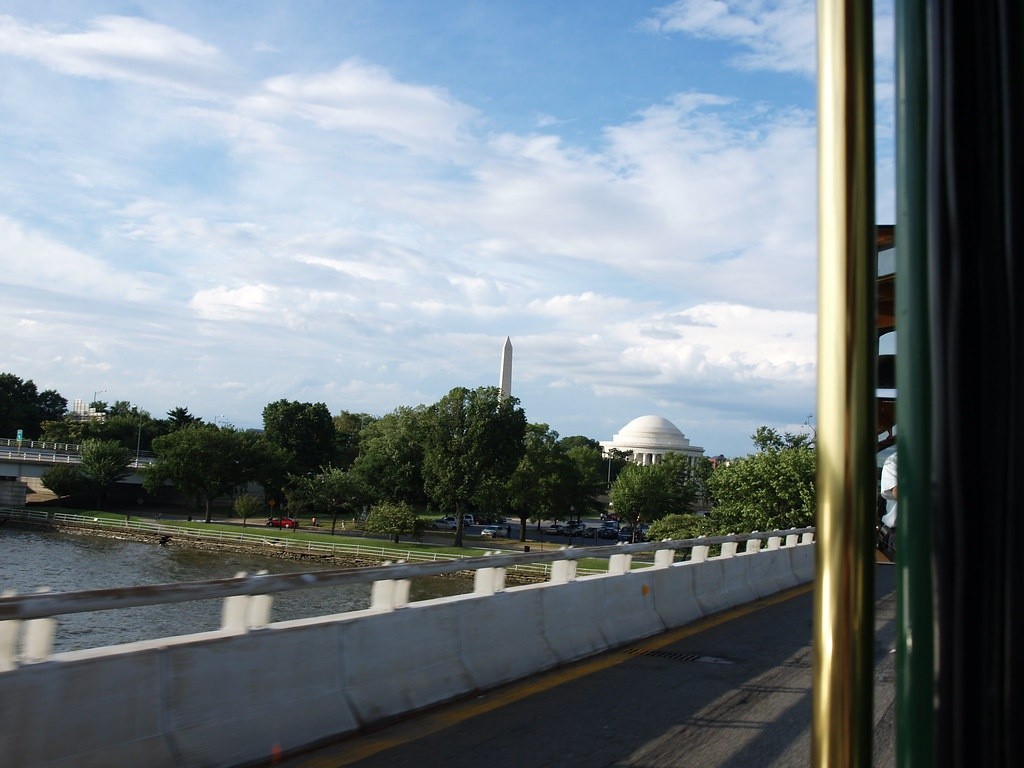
[
  {"xmin": 132, "ymin": 400, "xmax": 143, "ymax": 468},
  {"xmin": 94, "ymin": 390, "xmax": 106, "ymax": 403},
  {"xmin": 603, "ymin": 450, "xmax": 611, "ymax": 490}
]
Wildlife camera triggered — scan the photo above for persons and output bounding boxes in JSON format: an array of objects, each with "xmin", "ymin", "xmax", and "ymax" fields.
[
  {"xmin": 876, "ymin": 450, "xmax": 898, "ymax": 563},
  {"xmin": 312, "ymin": 516, "xmax": 317, "ymax": 526},
  {"xmin": 600, "ymin": 513, "xmax": 604, "ymax": 521},
  {"xmin": 507, "ymin": 525, "xmax": 512, "ymax": 538}
]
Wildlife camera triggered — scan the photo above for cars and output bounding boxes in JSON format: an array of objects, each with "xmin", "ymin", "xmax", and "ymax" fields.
[
  {"xmin": 443, "ymin": 517, "xmax": 456, "ymax": 524},
  {"xmin": 429, "ymin": 518, "xmax": 457, "ymax": 531},
  {"xmin": 267, "ymin": 517, "xmax": 299, "ymax": 528},
  {"xmin": 463, "ymin": 514, "xmax": 473, "ymax": 526},
  {"xmin": 545, "ymin": 525, "xmax": 564, "ymax": 534},
  {"xmin": 599, "ymin": 513, "xmax": 622, "ymax": 522},
  {"xmin": 563, "ymin": 520, "xmax": 649, "ymax": 542},
  {"xmin": 481, "ymin": 526, "xmax": 507, "ymax": 539}
]
